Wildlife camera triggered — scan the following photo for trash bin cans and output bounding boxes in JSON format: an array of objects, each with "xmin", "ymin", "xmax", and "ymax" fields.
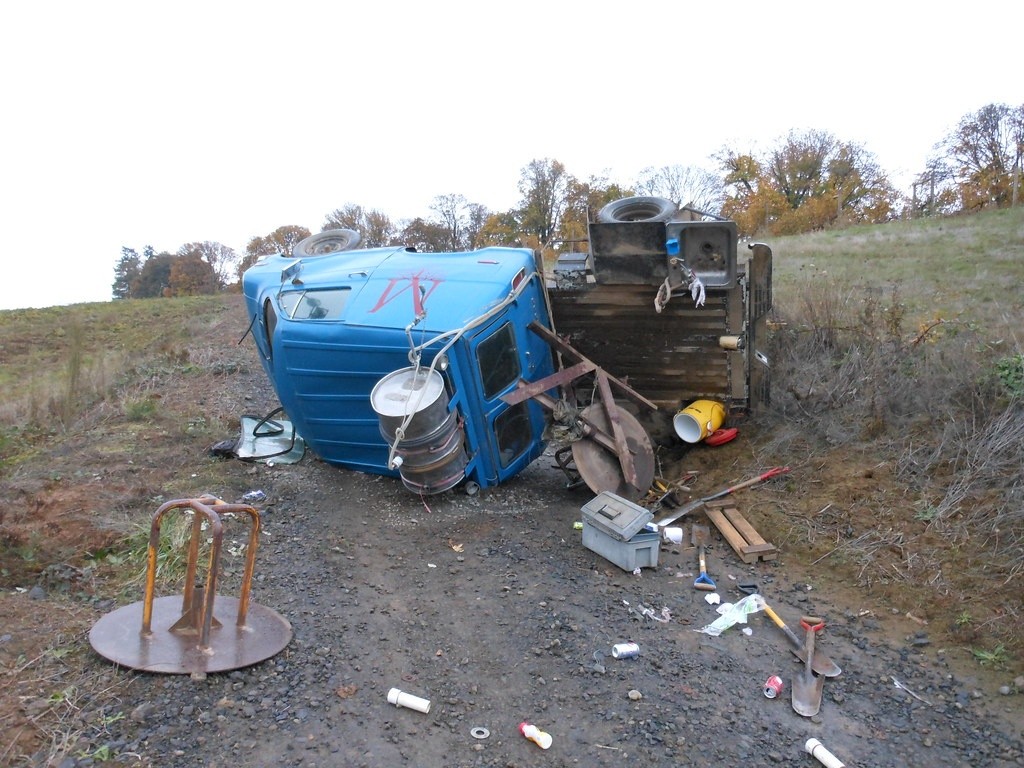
[{"xmin": 643, "ymin": 471, "xmax": 701, "ymax": 514}]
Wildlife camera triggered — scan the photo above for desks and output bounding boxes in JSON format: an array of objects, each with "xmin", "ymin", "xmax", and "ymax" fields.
[{"xmin": 89, "ymin": 493, "xmax": 293, "ymax": 679}]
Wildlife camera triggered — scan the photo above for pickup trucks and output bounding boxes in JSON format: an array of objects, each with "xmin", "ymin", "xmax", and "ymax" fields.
[{"xmin": 241, "ymin": 194, "xmax": 775, "ymax": 498}]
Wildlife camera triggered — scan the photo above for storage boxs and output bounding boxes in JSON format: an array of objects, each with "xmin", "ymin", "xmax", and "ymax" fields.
[{"xmin": 579, "ymin": 490, "xmax": 662, "ymax": 573}]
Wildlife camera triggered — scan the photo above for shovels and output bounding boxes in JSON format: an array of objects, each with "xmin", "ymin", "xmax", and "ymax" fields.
[
  {"xmin": 735, "ymin": 583, "xmax": 842, "ymax": 677},
  {"xmin": 791, "ymin": 616, "xmax": 825, "ymax": 717},
  {"xmin": 691, "ymin": 524, "xmax": 716, "ymax": 590},
  {"xmin": 657, "ymin": 466, "xmax": 791, "ymax": 526}
]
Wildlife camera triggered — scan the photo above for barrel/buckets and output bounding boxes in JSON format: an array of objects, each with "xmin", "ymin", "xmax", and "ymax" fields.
[
  {"xmin": 370, "ymin": 365, "xmax": 471, "ymax": 494},
  {"xmin": 672, "ymin": 400, "xmax": 726, "ymax": 444}
]
